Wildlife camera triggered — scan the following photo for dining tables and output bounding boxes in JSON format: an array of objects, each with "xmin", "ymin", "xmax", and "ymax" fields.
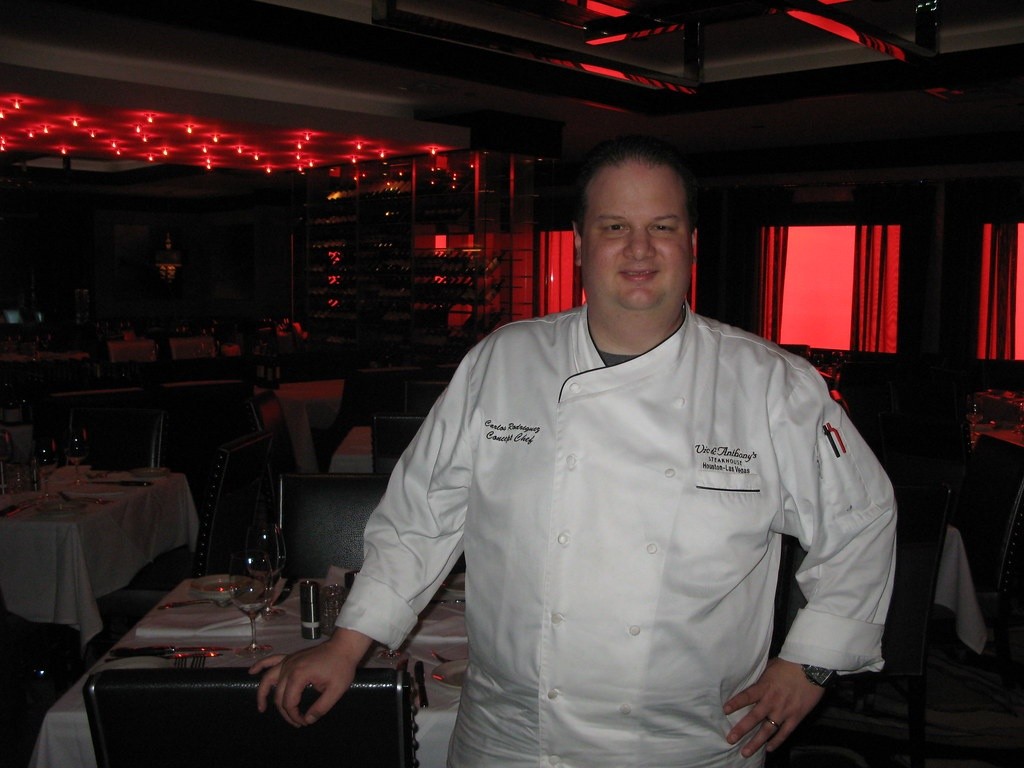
[
  {"xmin": 0, "ymin": 341, "xmax": 90, "ymax": 363},
  {"xmin": 0, "ymin": 462, "xmax": 202, "ymax": 660},
  {"xmin": 106, "ymin": 338, "xmax": 156, "ymax": 364},
  {"xmin": 252, "ymin": 380, "xmax": 345, "ymax": 430},
  {"xmin": 169, "ymin": 336, "xmax": 221, "ymax": 358},
  {"xmin": 30, "ymin": 573, "xmax": 471, "ymax": 768}
]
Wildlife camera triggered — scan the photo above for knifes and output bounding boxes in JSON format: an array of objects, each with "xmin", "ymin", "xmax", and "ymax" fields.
[
  {"xmin": 414, "ymin": 661, "xmax": 428, "ymax": 708},
  {"xmin": 104, "ymin": 645, "xmax": 231, "ymax": 661},
  {"xmin": 91, "ymin": 480, "xmax": 152, "ymax": 487}
]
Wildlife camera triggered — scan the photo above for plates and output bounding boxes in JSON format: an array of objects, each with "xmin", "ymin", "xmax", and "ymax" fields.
[
  {"xmin": 432, "ymin": 660, "xmax": 468, "ymax": 690},
  {"xmin": 190, "ymin": 574, "xmax": 252, "ymax": 594},
  {"xmin": 441, "ymin": 571, "xmax": 465, "ymax": 595},
  {"xmin": 32, "ymin": 502, "xmax": 88, "ymax": 517},
  {"xmin": 130, "ymin": 466, "xmax": 171, "ymax": 479}
]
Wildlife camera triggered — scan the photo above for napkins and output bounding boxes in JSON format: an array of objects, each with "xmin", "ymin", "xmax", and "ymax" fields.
[{"xmin": 137, "ymin": 602, "xmax": 263, "ymax": 637}]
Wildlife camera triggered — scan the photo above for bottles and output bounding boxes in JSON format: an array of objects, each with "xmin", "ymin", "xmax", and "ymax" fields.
[{"xmin": 306, "ymin": 164, "xmax": 508, "ymax": 358}]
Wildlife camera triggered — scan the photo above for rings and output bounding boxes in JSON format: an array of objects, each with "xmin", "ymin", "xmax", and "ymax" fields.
[{"xmin": 765, "ymin": 717, "xmax": 780, "ymax": 728}]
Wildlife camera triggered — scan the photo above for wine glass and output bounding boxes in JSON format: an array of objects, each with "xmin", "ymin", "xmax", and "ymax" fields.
[
  {"xmin": 964, "ymin": 394, "xmax": 983, "ymax": 439},
  {"xmin": 228, "ymin": 551, "xmax": 272, "ymax": 657},
  {"xmin": 24, "ymin": 427, "xmax": 87, "ymax": 503},
  {"xmin": 245, "ymin": 523, "xmax": 286, "ymax": 620}
]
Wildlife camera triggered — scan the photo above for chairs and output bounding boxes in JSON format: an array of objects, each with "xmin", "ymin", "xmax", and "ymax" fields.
[{"xmin": 0, "ymin": 317, "xmax": 1024, "ymax": 767}]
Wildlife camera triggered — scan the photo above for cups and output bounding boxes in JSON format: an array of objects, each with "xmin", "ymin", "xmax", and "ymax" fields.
[
  {"xmin": 320, "ymin": 584, "xmax": 347, "ymax": 637},
  {"xmin": 299, "ymin": 580, "xmax": 320, "ymax": 639}
]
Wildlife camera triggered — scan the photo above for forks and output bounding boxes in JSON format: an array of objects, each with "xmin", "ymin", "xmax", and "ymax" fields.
[
  {"xmin": 157, "ymin": 598, "xmax": 233, "ymax": 608},
  {"xmin": 190, "ymin": 655, "xmax": 206, "ymax": 668},
  {"xmin": 173, "ymin": 657, "xmax": 187, "ymax": 668}
]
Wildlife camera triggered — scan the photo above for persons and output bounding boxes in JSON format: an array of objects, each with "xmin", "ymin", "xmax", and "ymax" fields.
[{"xmin": 247, "ymin": 133, "xmax": 898, "ymax": 768}]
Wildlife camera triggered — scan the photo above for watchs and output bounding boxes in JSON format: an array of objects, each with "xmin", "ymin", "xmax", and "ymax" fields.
[{"xmin": 803, "ymin": 665, "xmax": 834, "ymax": 686}]
